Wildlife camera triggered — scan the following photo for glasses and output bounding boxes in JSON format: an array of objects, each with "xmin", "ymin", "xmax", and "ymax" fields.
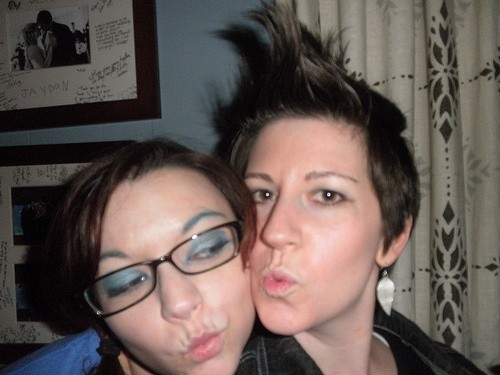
[{"xmin": 82, "ymin": 220, "xmax": 242, "ymax": 318}]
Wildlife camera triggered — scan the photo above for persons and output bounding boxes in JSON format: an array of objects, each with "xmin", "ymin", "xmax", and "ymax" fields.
[
  {"xmin": 14, "ymin": 41, "xmax": 28, "ymax": 70},
  {"xmin": 22, "ymin": 22, "xmax": 55, "ymax": 69},
  {"xmin": 83, "ymin": 22, "xmax": 90, "ymax": 56},
  {"xmin": 37, "ymin": 10, "xmax": 78, "ymax": 68},
  {"xmin": 217, "ymin": 0, "xmax": 486, "ymax": 375},
  {"xmin": 32, "ymin": 138, "xmax": 259, "ymax": 375},
  {"xmin": 70, "ymin": 21, "xmax": 89, "ymax": 64}
]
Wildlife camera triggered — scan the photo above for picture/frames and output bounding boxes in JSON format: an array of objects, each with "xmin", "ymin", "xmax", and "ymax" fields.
[
  {"xmin": 0, "ymin": 0, "xmax": 162, "ymax": 133},
  {"xmin": 0, "ymin": 140, "xmax": 142, "ymax": 366}
]
